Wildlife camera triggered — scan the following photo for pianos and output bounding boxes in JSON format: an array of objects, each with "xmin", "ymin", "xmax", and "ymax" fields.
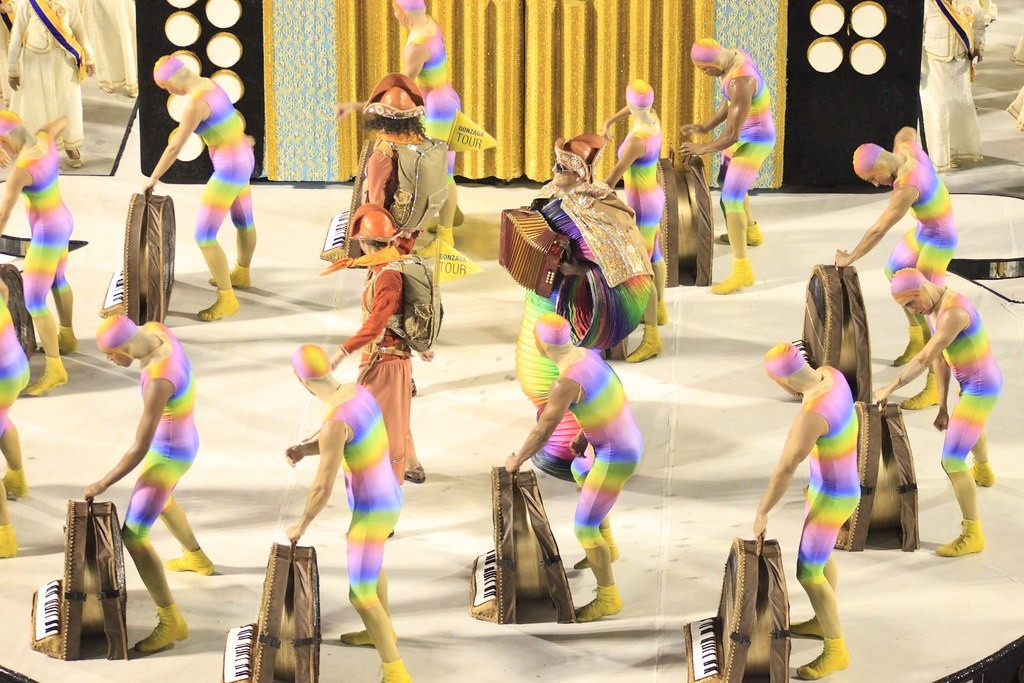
[
  {"xmin": 789, "ymin": 264, "xmax": 872, "ymax": 404},
  {"xmin": 222, "ymin": 544, "xmax": 322, "ymax": 683},
  {"xmin": 803, "ymin": 401, "xmax": 920, "ymax": 552},
  {"xmin": 99, "ymin": 191, "xmax": 176, "ymax": 326},
  {"xmin": 656, "ymin": 145, "xmax": 712, "ymax": 288},
  {"xmin": 684, "ymin": 539, "xmax": 791, "ymax": 683},
  {"xmin": 320, "ymin": 140, "xmax": 375, "ymax": 268},
  {"xmin": 31, "ymin": 500, "xmax": 129, "ymax": 660},
  {"xmin": 468, "ymin": 468, "xmax": 577, "ymax": 624},
  {"xmin": 0, "ymin": 264, "xmax": 38, "ymax": 361}
]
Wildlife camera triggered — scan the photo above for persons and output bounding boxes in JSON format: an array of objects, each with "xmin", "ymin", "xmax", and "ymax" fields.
[
  {"xmin": 0, "ymin": 110, "xmax": 78, "ymax": 395},
  {"xmin": 142, "ymin": 54, "xmax": 259, "ymax": 324},
  {"xmin": 753, "ymin": 0, "xmax": 1024, "ymax": 683},
  {"xmin": 286, "ymin": 0, "xmax": 497, "ymax": 681},
  {"xmin": 84, "ymin": 315, "xmax": 215, "ymax": 652},
  {"xmin": 0, "ymin": 1, "xmax": 138, "ymax": 167},
  {"xmin": 0, "ymin": 277, "xmax": 31, "ymax": 556},
  {"xmin": 503, "ymin": 38, "xmax": 777, "ymax": 622}
]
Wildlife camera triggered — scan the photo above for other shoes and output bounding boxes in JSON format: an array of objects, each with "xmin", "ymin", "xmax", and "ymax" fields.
[
  {"xmin": 341, "ymin": 615, "xmax": 397, "ymax": 646},
  {"xmin": 936, "ymin": 519, "xmax": 985, "ymax": 557},
  {"xmin": 1, "ymin": 467, "xmax": 28, "ymax": 499},
  {"xmin": 134, "ymin": 602, "xmax": 189, "ymax": 652},
  {"xmin": 404, "ymin": 463, "xmax": 426, "ymax": 483},
  {"xmin": 165, "ymin": 547, "xmax": 215, "ymax": 576},
  {"xmin": 894, "ymin": 326, "xmax": 938, "ymax": 410},
  {"xmin": 572, "ymin": 529, "xmax": 619, "ymax": 571},
  {"xmin": 27, "ymin": 325, "xmax": 78, "ymax": 395},
  {"xmin": 790, "ymin": 615, "xmax": 850, "ymax": 680},
  {"xmin": 197, "ymin": 262, "xmax": 251, "ymax": 321},
  {"xmin": 712, "ymin": 221, "xmax": 763, "ymax": 294},
  {"xmin": 627, "ymin": 303, "xmax": 668, "ymax": 362},
  {"xmin": 0, "ymin": 523, "xmax": 18, "ymax": 557},
  {"xmin": 410, "ymin": 379, "xmax": 417, "ymax": 396},
  {"xmin": 969, "ymin": 458, "xmax": 995, "ymax": 487},
  {"xmin": 378, "ymin": 659, "xmax": 413, "ymax": 683},
  {"xmin": 574, "ymin": 583, "xmax": 623, "ymax": 622}
]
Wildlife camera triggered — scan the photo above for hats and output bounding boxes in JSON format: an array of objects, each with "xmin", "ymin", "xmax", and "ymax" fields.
[
  {"xmin": 351, "ymin": 211, "xmax": 401, "ymax": 241},
  {"xmin": 363, "ymin": 74, "xmax": 428, "ymax": 120},
  {"xmin": 555, "ymin": 133, "xmax": 607, "ymax": 185}
]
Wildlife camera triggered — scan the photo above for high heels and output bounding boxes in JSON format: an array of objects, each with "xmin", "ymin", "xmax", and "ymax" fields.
[{"xmin": 66, "ymin": 149, "xmax": 82, "ymax": 167}]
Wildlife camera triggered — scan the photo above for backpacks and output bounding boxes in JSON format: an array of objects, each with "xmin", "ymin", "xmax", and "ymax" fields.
[
  {"xmin": 375, "ymin": 254, "xmax": 444, "ymax": 353},
  {"xmin": 373, "ymin": 136, "xmax": 449, "ymax": 228}
]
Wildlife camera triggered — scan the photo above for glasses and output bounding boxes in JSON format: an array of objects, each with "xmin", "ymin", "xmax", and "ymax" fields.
[{"xmin": 554, "ymin": 159, "xmax": 570, "ymax": 175}]
